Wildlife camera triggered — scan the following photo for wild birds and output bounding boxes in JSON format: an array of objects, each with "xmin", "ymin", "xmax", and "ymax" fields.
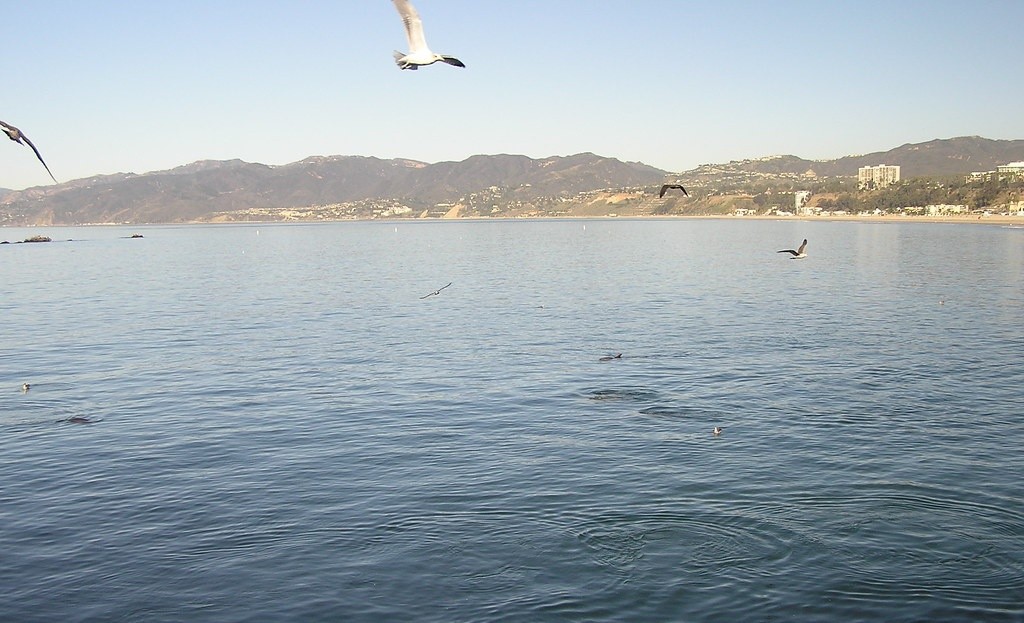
[
  {"xmin": 389, "ymin": 0, "xmax": 465, "ymax": 71},
  {"xmin": 419, "ymin": 282, "xmax": 452, "ymax": 300},
  {"xmin": 939, "ymin": 300, "xmax": 946, "ymax": 305},
  {"xmin": 776, "ymin": 239, "xmax": 809, "ymax": 260},
  {"xmin": 658, "ymin": 184, "xmax": 689, "ymax": 200},
  {"xmin": 713, "ymin": 425, "xmax": 722, "ymax": 435},
  {"xmin": 23, "ymin": 382, "xmax": 30, "ymax": 390},
  {"xmin": 0, "ymin": 120, "xmax": 59, "ymax": 186}
]
